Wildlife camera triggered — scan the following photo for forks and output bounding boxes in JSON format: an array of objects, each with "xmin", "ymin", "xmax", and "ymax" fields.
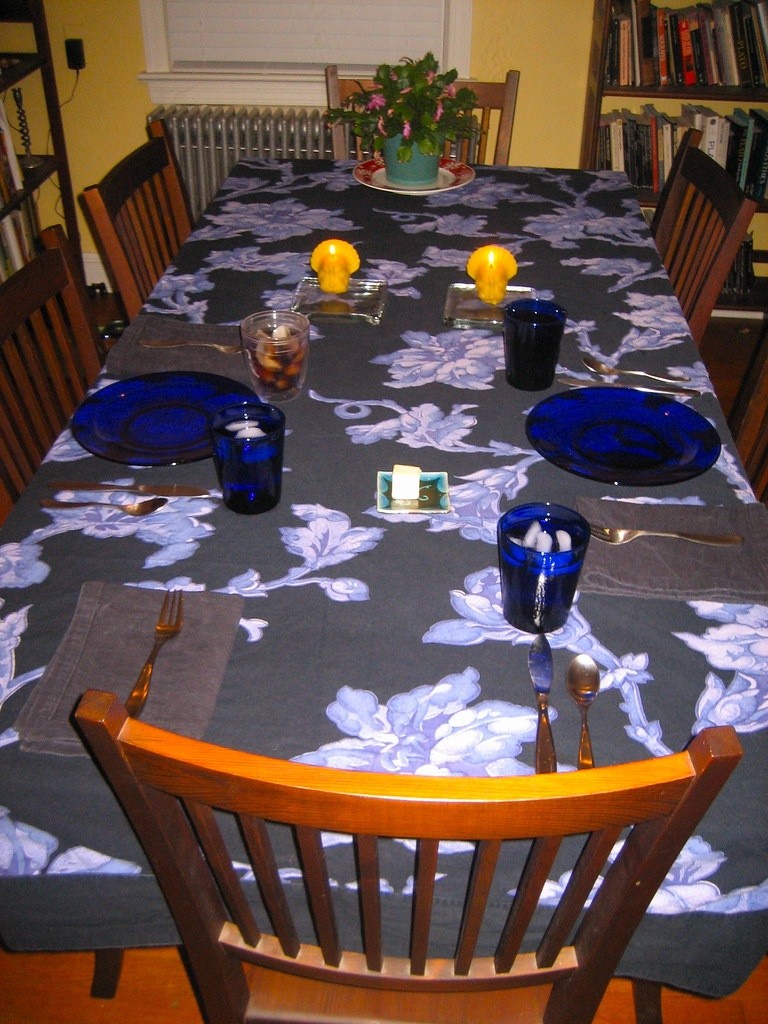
[
  {"xmin": 124, "ymin": 588, "xmax": 183, "ymax": 717},
  {"xmin": 138, "ymin": 339, "xmax": 243, "ymax": 355},
  {"xmin": 589, "ymin": 521, "xmax": 744, "ymax": 548}
]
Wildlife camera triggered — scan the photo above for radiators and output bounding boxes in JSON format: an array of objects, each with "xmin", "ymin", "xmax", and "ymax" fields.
[{"xmin": 146, "ymin": 104, "xmax": 483, "ymax": 228}]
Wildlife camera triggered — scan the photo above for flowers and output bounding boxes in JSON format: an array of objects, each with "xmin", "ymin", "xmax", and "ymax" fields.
[{"xmin": 329, "ymin": 50, "xmax": 489, "ymax": 162}]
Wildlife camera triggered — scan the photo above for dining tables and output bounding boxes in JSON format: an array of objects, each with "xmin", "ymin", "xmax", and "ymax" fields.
[{"xmin": 0, "ymin": 151, "xmax": 768, "ymax": 1024}]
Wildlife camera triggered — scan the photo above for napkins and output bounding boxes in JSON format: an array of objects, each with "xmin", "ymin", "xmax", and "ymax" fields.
[
  {"xmin": 12, "ymin": 580, "xmax": 245, "ymax": 760},
  {"xmin": 571, "ymin": 495, "xmax": 768, "ymax": 607},
  {"xmin": 106, "ymin": 315, "xmax": 256, "ymax": 391}
]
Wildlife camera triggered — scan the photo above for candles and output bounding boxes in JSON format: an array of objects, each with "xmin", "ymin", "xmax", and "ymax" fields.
[
  {"xmin": 476, "ymin": 251, "xmax": 509, "ymax": 304},
  {"xmin": 318, "ymin": 244, "xmax": 350, "ymax": 294}
]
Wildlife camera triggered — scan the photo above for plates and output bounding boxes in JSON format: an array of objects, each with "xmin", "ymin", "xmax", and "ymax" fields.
[
  {"xmin": 352, "ymin": 157, "xmax": 476, "ymax": 197},
  {"xmin": 71, "ymin": 370, "xmax": 262, "ymax": 466},
  {"xmin": 524, "ymin": 386, "xmax": 722, "ymax": 488}
]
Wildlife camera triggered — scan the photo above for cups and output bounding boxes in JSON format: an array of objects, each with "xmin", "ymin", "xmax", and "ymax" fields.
[
  {"xmin": 497, "ymin": 501, "xmax": 591, "ymax": 635},
  {"xmin": 503, "ymin": 298, "xmax": 568, "ymax": 392},
  {"xmin": 209, "ymin": 403, "xmax": 286, "ymax": 514},
  {"xmin": 240, "ymin": 309, "xmax": 310, "ymax": 404}
]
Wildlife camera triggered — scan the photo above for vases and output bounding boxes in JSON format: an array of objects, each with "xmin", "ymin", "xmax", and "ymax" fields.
[{"xmin": 382, "ymin": 133, "xmax": 439, "ymax": 186}]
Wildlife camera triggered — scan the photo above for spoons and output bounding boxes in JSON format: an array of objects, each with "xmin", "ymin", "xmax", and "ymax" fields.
[
  {"xmin": 566, "ymin": 653, "xmax": 601, "ymax": 771},
  {"xmin": 39, "ymin": 497, "xmax": 168, "ymax": 516},
  {"xmin": 581, "ymin": 357, "xmax": 691, "ymax": 383}
]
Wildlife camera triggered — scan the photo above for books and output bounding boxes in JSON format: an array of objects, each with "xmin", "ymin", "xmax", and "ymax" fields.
[
  {"xmin": 0, "ymin": 98, "xmax": 38, "ymax": 283},
  {"xmin": 597, "ymin": 1, "xmax": 768, "ymax": 307}
]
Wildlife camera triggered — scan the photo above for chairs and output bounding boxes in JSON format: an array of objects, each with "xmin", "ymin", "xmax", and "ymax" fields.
[
  {"xmin": 649, "ymin": 128, "xmax": 758, "ymax": 348},
  {"xmin": 725, "ymin": 321, "xmax": 768, "ymax": 503},
  {"xmin": 74, "ymin": 687, "xmax": 743, "ymax": 1024},
  {"xmin": 326, "ymin": 65, "xmax": 521, "ymax": 166},
  {"xmin": 84, "ymin": 119, "xmax": 194, "ymax": 323},
  {"xmin": 0, "ymin": 224, "xmax": 105, "ymax": 525}
]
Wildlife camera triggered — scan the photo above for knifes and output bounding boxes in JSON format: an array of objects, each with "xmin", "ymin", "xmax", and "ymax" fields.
[
  {"xmin": 557, "ymin": 378, "xmax": 700, "ymax": 398},
  {"xmin": 527, "ymin": 633, "xmax": 557, "ymax": 774},
  {"xmin": 46, "ymin": 479, "xmax": 209, "ymax": 497}
]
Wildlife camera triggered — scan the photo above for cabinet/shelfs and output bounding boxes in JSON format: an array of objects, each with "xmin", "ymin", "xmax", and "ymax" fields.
[
  {"xmin": 579, "ymin": 0, "xmax": 768, "ymax": 214},
  {"xmin": 0, "ymin": 0, "xmax": 83, "ymax": 276}
]
